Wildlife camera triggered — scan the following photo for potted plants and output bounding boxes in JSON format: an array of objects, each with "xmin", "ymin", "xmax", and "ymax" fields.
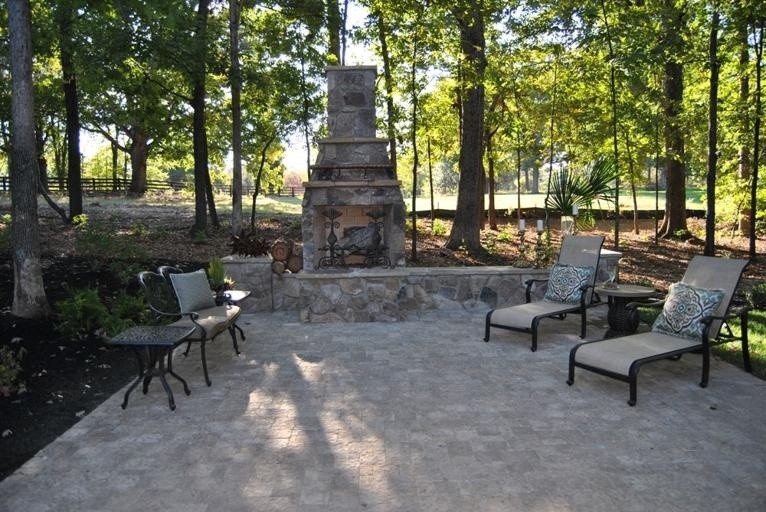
[{"xmin": 207, "ymin": 253, "xmax": 227, "ymax": 294}]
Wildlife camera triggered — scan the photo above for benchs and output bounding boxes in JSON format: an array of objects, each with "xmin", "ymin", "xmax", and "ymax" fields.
[{"xmin": 136, "ymin": 266, "xmax": 246, "ymax": 386}]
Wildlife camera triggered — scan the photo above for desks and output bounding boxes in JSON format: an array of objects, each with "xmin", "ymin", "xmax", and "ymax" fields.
[
  {"xmin": 105, "ymin": 325, "xmax": 197, "ymax": 410},
  {"xmin": 212, "ymin": 289, "xmax": 252, "ymax": 348}
]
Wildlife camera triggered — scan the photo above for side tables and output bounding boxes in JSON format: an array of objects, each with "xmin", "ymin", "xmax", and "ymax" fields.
[{"xmin": 594, "ymin": 282, "xmax": 656, "ymax": 342}]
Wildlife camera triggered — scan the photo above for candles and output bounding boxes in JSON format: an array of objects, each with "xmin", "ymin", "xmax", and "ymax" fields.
[
  {"xmin": 519, "ymin": 219, "xmax": 525, "ymax": 232},
  {"xmin": 537, "ymin": 219, "xmax": 544, "ymax": 232}
]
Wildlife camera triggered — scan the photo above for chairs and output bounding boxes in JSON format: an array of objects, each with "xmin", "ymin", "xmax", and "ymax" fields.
[
  {"xmin": 565, "ymin": 255, "xmax": 751, "ymax": 406},
  {"xmin": 483, "ymin": 235, "xmax": 606, "ymax": 351}
]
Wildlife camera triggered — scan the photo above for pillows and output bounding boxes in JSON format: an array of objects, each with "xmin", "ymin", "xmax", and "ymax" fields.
[
  {"xmin": 650, "ymin": 281, "xmax": 725, "ymax": 342},
  {"xmin": 542, "ymin": 262, "xmax": 592, "ymax": 305}
]
